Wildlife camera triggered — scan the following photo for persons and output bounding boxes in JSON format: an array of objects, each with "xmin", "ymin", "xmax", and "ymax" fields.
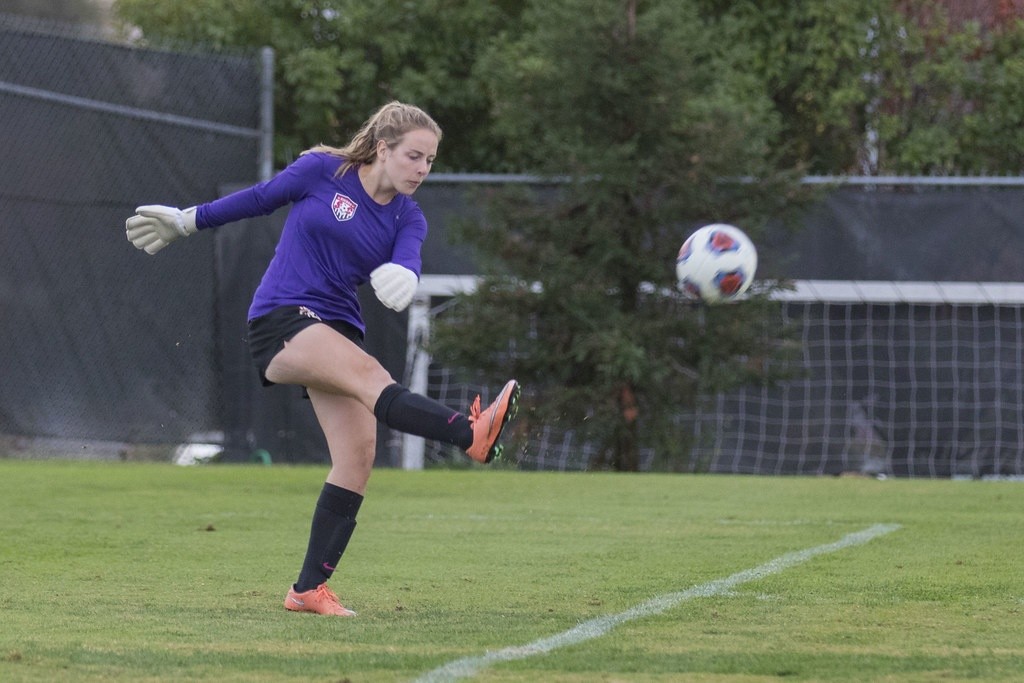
[
  {"xmin": 844, "ymin": 390, "xmax": 888, "ymax": 476},
  {"xmin": 126, "ymin": 100, "xmax": 522, "ymax": 615}
]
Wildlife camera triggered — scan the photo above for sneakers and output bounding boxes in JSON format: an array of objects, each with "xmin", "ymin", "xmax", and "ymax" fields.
[
  {"xmin": 283, "ymin": 582, "xmax": 356, "ymax": 617},
  {"xmin": 466, "ymin": 379, "xmax": 522, "ymax": 463}
]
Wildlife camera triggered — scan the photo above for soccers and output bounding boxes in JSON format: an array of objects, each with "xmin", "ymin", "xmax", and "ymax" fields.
[{"xmin": 678, "ymin": 222, "xmax": 759, "ymax": 306}]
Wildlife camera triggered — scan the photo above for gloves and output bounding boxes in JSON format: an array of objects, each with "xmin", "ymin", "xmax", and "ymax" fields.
[
  {"xmin": 370, "ymin": 262, "xmax": 417, "ymax": 313},
  {"xmin": 126, "ymin": 201, "xmax": 198, "ymax": 256}
]
